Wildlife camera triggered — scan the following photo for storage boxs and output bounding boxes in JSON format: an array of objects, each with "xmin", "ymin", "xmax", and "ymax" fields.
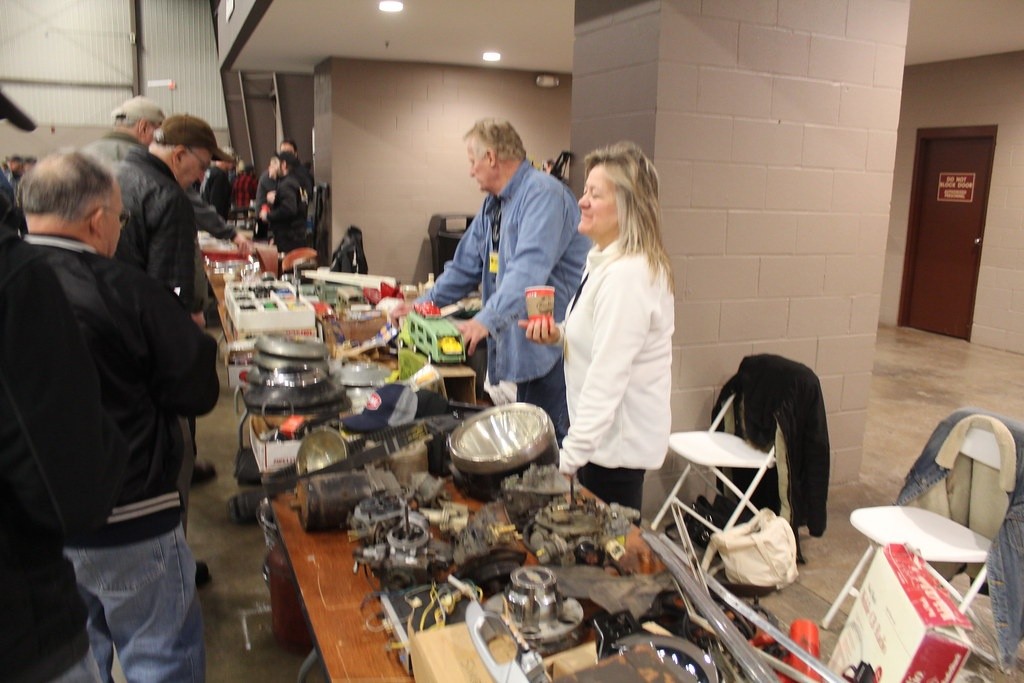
[{"xmin": 826, "ymin": 542, "xmax": 973, "ymax": 682}]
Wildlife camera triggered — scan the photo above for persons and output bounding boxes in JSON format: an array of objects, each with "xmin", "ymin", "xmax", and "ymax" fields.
[
  {"xmin": 0, "ymin": 91, "xmax": 102, "ymax": 683},
  {"xmin": 2, "ymin": 152, "xmax": 40, "ymax": 190},
  {"xmin": 77, "ymin": 94, "xmax": 255, "ymax": 488},
  {"xmin": 202, "ymin": 136, "xmax": 321, "ymax": 257},
  {"xmin": 104, "ymin": 111, "xmax": 235, "ymax": 590},
  {"xmin": 412, "ymin": 117, "xmax": 590, "ymax": 464},
  {"xmin": 516, "ymin": 140, "xmax": 672, "ymax": 529},
  {"xmin": 0, "ymin": 151, "xmax": 225, "ymax": 683}
]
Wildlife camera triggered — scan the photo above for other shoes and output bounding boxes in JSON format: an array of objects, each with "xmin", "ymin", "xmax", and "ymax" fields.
[
  {"xmin": 190, "ymin": 456, "xmax": 217, "ymax": 485},
  {"xmin": 194, "ymin": 559, "xmax": 213, "ymax": 588}
]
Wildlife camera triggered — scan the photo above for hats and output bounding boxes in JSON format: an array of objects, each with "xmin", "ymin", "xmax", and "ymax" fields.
[
  {"xmin": 154, "ymin": 113, "xmax": 236, "ymax": 162},
  {"xmin": 339, "ymin": 382, "xmax": 419, "ymax": 433},
  {"xmin": 111, "ymin": 95, "xmax": 167, "ymax": 127},
  {"xmin": 274, "ymin": 151, "xmax": 300, "ymax": 168}
]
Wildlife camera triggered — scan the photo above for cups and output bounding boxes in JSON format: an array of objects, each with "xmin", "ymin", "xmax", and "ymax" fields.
[{"xmin": 526, "ymin": 285, "xmax": 556, "ymax": 336}]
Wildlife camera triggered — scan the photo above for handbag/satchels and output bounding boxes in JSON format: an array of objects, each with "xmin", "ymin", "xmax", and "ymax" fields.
[
  {"xmin": 686, "ymin": 495, "xmax": 721, "ymax": 558},
  {"xmin": 710, "ymin": 508, "xmax": 800, "ymax": 590}
]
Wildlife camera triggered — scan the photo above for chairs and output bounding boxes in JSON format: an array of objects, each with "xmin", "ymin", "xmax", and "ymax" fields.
[
  {"xmin": 820, "ymin": 407, "xmax": 1023, "ymax": 683},
  {"xmin": 649, "ymin": 353, "xmax": 821, "ymax": 574}
]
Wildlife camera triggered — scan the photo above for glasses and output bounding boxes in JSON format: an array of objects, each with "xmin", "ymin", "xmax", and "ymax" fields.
[
  {"xmin": 103, "ymin": 205, "xmax": 131, "ymax": 231},
  {"xmin": 184, "ymin": 145, "xmax": 209, "ymax": 171}
]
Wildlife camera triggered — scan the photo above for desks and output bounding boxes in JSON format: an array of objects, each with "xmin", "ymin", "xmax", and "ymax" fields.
[{"xmin": 194, "ymin": 229, "xmax": 742, "ymax": 682}]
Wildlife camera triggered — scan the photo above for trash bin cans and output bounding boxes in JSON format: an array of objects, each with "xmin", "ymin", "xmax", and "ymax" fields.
[{"xmin": 428, "ymin": 213, "xmax": 476, "ymax": 284}]
